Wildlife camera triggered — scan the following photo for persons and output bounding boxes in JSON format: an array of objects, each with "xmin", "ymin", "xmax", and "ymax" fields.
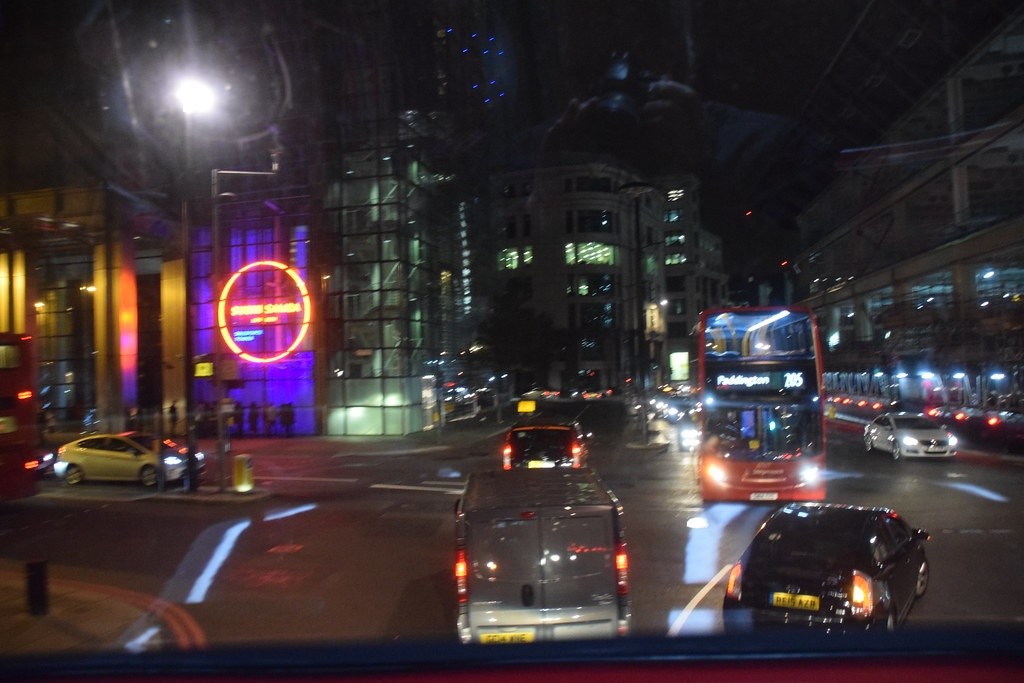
[{"xmin": 34, "ymin": 399, "xmax": 296, "ymax": 441}]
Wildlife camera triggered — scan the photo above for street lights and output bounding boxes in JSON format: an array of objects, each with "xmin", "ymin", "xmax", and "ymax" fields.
[
  {"xmin": 620, "ymin": 176, "xmax": 662, "ymax": 443},
  {"xmin": 192, "ymin": 350, "xmax": 244, "ymax": 488}
]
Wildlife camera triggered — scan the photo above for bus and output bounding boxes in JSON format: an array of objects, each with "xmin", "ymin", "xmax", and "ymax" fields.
[
  {"xmin": 690, "ymin": 300, "xmax": 829, "ymax": 505},
  {"xmin": 1, "ymin": 331, "xmax": 41, "ymax": 502}
]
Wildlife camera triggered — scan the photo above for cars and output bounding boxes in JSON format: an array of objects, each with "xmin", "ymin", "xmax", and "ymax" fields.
[
  {"xmin": 56, "ymin": 432, "xmax": 206, "ymax": 488},
  {"xmin": 829, "ymin": 394, "xmax": 1022, "ymax": 446},
  {"xmin": 862, "ymin": 409, "xmax": 963, "ymax": 462},
  {"xmin": 721, "ymin": 502, "xmax": 932, "ymax": 644},
  {"xmin": 443, "ymin": 363, "xmax": 700, "ymax": 423},
  {"xmin": 37, "ymin": 444, "xmax": 54, "ymax": 479}
]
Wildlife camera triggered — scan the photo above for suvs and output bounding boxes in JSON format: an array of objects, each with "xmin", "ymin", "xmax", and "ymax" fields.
[{"xmin": 504, "ymin": 414, "xmax": 594, "ymax": 468}]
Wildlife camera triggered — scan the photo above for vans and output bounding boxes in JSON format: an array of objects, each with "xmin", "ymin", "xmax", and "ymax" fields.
[{"xmin": 454, "ymin": 464, "xmax": 631, "ymax": 644}]
